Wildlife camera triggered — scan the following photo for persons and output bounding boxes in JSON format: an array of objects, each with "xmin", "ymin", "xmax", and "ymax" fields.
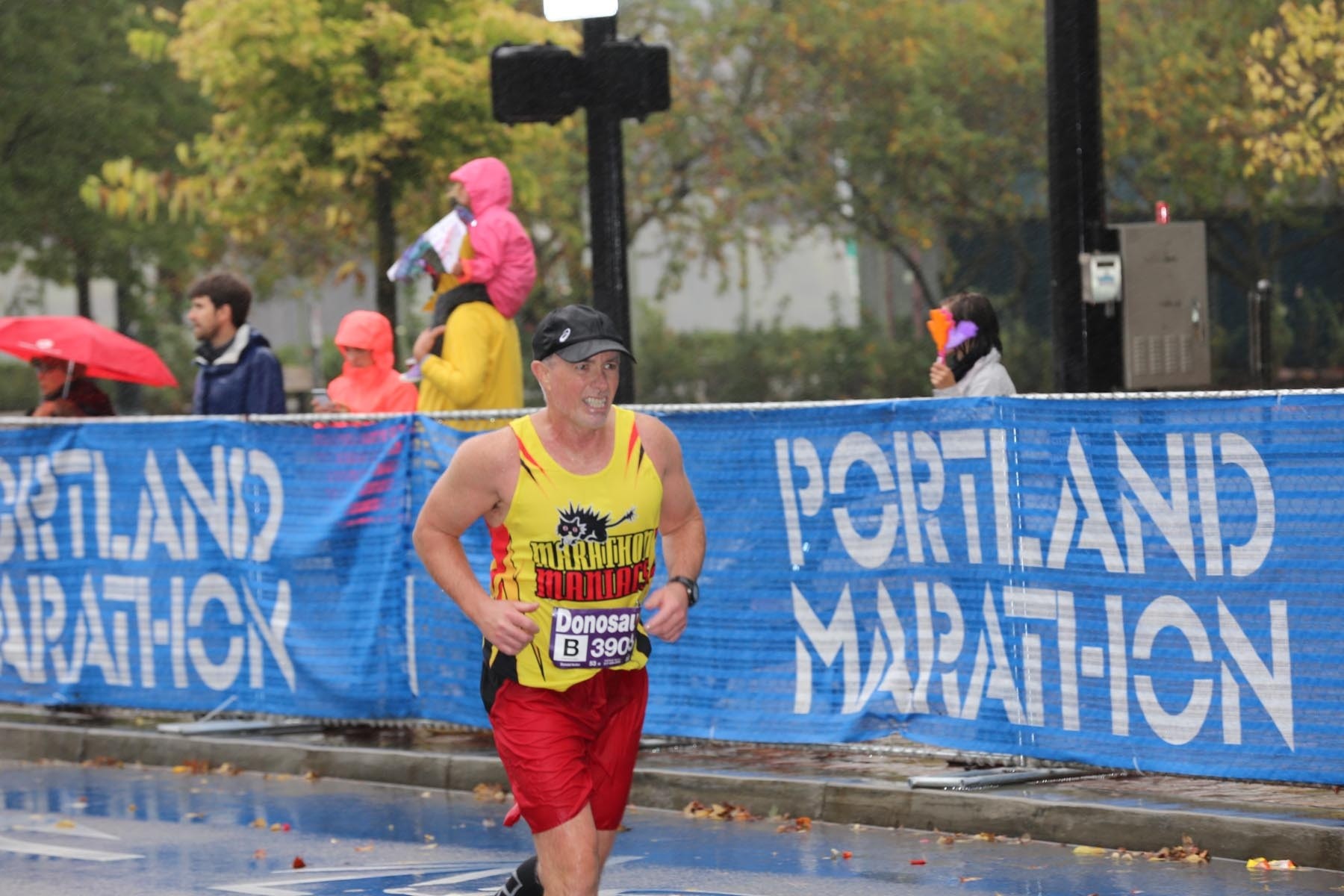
[
  {"xmin": 926, "ymin": 293, "xmax": 1017, "ymax": 396},
  {"xmin": 309, "ymin": 310, "xmax": 419, "ymax": 413},
  {"xmin": 20, "ymin": 354, "xmax": 117, "ymax": 417},
  {"xmin": 413, "ymin": 304, "xmax": 706, "ymax": 896},
  {"xmin": 186, "ymin": 273, "xmax": 288, "ymax": 414},
  {"xmin": 385, "ymin": 157, "xmax": 535, "ymax": 412}
]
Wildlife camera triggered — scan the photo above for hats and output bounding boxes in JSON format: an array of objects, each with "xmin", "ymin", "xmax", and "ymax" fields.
[
  {"xmin": 28, "ymin": 355, "xmax": 86, "ymax": 380},
  {"xmin": 531, "ymin": 303, "xmax": 638, "ymax": 364}
]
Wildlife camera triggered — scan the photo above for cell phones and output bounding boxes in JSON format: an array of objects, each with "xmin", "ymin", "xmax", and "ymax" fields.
[{"xmin": 312, "ymin": 389, "xmax": 331, "ymax": 404}]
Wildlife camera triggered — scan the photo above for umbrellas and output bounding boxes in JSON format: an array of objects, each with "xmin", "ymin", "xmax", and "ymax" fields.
[{"xmin": 0, "ymin": 315, "xmax": 180, "ymax": 400}]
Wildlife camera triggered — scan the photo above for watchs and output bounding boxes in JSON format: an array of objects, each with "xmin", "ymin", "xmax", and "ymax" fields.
[{"xmin": 669, "ymin": 575, "xmax": 700, "ymax": 608}]
